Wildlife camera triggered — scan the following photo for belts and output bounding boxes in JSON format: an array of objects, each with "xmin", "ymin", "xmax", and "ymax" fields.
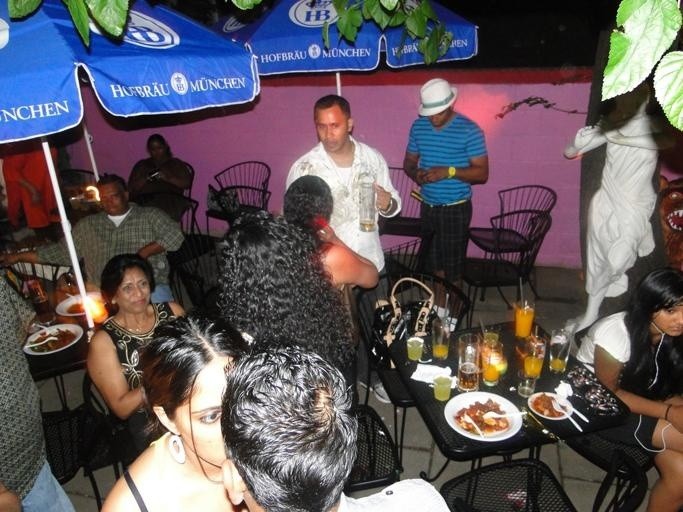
[{"xmin": 409, "ymin": 192, "xmax": 470, "ymax": 207}]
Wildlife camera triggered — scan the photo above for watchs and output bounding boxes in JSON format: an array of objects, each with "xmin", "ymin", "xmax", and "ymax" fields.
[{"xmin": 447, "ymin": 166, "xmax": 456, "ymax": 179}]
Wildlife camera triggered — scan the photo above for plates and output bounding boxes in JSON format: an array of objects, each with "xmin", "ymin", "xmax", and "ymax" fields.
[
  {"xmin": 444, "ymin": 391, "xmax": 523, "ymax": 443},
  {"xmin": 527, "ymin": 391, "xmax": 572, "ymax": 420},
  {"xmin": 23, "ymin": 323, "xmax": 84, "ymax": 355},
  {"xmin": 56, "ymin": 292, "xmax": 107, "ymax": 316}
]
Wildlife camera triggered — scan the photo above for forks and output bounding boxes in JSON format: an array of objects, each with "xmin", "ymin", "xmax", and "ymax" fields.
[
  {"xmin": 551, "ymin": 399, "xmax": 583, "ymax": 434},
  {"xmin": 461, "ymin": 412, "xmax": 487, "ymax": 440}
]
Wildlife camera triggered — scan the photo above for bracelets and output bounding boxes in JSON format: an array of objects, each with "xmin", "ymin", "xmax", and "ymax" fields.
[
  {"xmin": 664, "ymin": 403, "xmax": 675, "ymax": 421},
  {"xmin": 379, "ymin": 198, "xmax": 394, "ymax": 214}
]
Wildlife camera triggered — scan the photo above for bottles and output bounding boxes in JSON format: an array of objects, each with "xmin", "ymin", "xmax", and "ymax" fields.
[{"xmin": 28, "ymin": 276, "xmax": 50, "ymax": 314}]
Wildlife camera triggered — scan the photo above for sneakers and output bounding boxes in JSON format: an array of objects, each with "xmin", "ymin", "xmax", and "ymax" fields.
[{"xmin": 358, "ymin": 379, "xmax": 392, "ymax": 404}]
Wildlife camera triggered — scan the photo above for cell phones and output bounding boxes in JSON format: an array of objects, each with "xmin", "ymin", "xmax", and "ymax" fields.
[{"xmin": 147, "ymin": 169, "xmax": 159, "ymax": 178}]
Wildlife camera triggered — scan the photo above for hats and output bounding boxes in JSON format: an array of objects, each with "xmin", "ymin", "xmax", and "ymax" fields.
[{"xmin": 417, "ymin": 78, "xmax": 458, "ymax": 117}]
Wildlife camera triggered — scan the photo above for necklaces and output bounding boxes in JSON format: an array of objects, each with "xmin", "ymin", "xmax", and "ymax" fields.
[{"xmin": 118, "ymin": 312, "xmax": 146, "ymax": 333}]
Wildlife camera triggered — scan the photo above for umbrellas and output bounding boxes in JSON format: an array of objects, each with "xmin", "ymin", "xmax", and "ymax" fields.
[
  {"xmin": 0, "ymin": 0, "xmax": 259, "ymax": 328},
  {"xmin": 205, "ymin": 0, "xmax": 479, "ymax": 97}
]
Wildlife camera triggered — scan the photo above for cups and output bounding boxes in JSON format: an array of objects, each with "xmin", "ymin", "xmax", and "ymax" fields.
[
  {"xmin": 434, "ymin": 377, "xmax": 451, "ymax": 401},
  {"xmin": 431, "ymin": 316, "xmax": 448, "ymax": 360},
  {"xmin": 519, "ymin": 369, "xmax": 537, "ymax": 398},
  {"xmin": 458, "ymin": 334, "xmax": 480, "ymax": 393},
  {"xmin": 406, "ymin": 337, "xmax": 424, "ymax": 361},
  {"xmin": 358, "ymin": 173, "xmax": 381, "ymax": 231},
  {"xmin": 484, "ymin": 341, "xmax": 503, "ymax": 388},
  {"xmin": 524, "ymin": 336, "xmax": 547, "ymax": 379},
  {"xmin": 550, "ymin": 328, "xmax": 570, "ymax": 374},
  {"xmin": 514, "ymin": 301, "xmax": 535, "ymax": 340}
]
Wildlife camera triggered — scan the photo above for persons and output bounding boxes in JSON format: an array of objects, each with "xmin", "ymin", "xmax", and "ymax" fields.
[
  {"xmin": 84, "ymin": 252, "xmax": 188, "ymax": 455},
  {"xmin": 576, "ymin": 268, "xmax": 683, "ymax": 512},
  {"xmin": 564, "ymin": 80, "xmax": 678, "ymax": 338},
  {"xmin": 286, "ymin": 94, "xmax": 402, "ymax": 404},
  {"xmin": 220, "ymin": 336, "xmax": 453, "ymax": 512},
  {"xmin": 1, "ymin": 132, "xmax": 78, "ymax": 241},
  {"xmin": 127, "ymin": 133, "xmax": 193, "ymax": 224},
  {"xmin": 0, "ymin": 173, "xmax": 186, "ymax": 316},
  {"xmin": 216, "ymin": 210, "xmax": 361, "ymax": 408},
  {"xmin": 0, "ymin": 273, "xmax": 78, "ymax": 512},
  {"xmin": 282, "ymin": 173, "xmax": 379, "ymax": 348},
  {"xmin": 404, "ymin": 78, "xmax": 490, "ymax": 317},
  {"xmin": 100, "ymin": 304, "xmax": 252, "ymax": 511}
]
